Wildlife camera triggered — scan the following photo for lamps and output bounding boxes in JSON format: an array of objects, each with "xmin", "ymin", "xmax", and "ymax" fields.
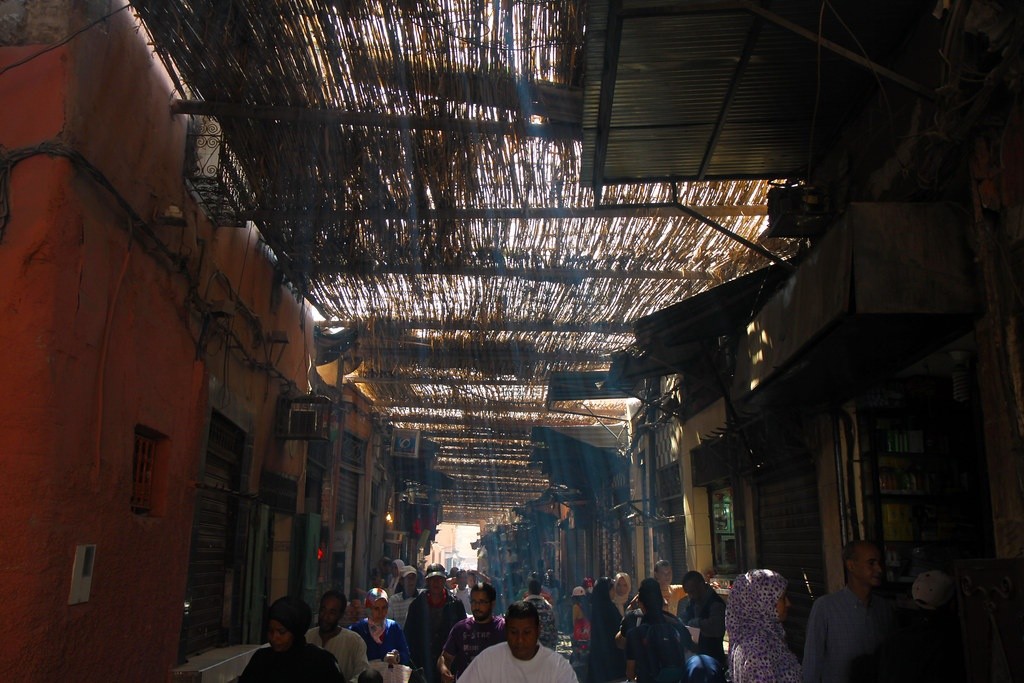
[
  {"xmin": 768, "ymin": 177, "xmax": 833, "ymax": 240},
  {"xmin": 153, "ymin": 201, "xmax": 188, "ymax": 229},
  {"xmin": 268, "ymin": 329, "xmax": 290, "ymax": 365}
]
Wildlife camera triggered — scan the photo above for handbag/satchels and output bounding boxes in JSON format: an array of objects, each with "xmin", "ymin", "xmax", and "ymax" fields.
[{"xmin": 371, "ymin": 661, "xmax": 411, "ymax": 683}]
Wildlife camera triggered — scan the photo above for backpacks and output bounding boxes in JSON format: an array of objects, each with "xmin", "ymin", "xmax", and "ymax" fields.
[{"xmin": 636, "ymin": 616, "xmax": 686, "ymax": 683}]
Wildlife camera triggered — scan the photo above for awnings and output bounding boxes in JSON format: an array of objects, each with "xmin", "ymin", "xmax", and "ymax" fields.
[
  {"xmin": 548, "ymin": 370, "xmax": 685, "ymax": 420},
  {"xmin": 528, "ymin": 447, "xmax": 649, "ymax": 520},
  {"xmin": 602, "ymin": 253, "xmax": 805, "ymax": 472},
  {"xmin": 512, "ymin": 506, "xmax": 558, "ymax": 525},
  {"xmin": 579, "ymin": 0, "xmax": 954, "ymax": 184},
  {"xmin": 531, "ymin": 426, "xmax": 627, "ymax": 463}
]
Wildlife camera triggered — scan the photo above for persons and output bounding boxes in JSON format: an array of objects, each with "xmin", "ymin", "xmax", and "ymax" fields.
[
  {"xmin": 238, "ymin": 589, "xmax": 384, "ymax": 683},
  {"xmin": 386, "ymin": 559, "xmax": 405, "ymax": 596},
  {"xmin": 387, "ymin": 565, "xmax": 427, "ymax": 629},
  {"xmin": 802, "ymin": 542, "xmax": 965, "ymax": 683},
  {"xmin": 403, "ymin": 565, "xmax": 506, "ymax": 683},
  {"xmin": 615, "ymin": 558, "xmax": 803, "ymax": 683},
  {"xmin": 347, "ymin": 587, "xmax": 420, "ymax": 683},
  {"xmin": 455, "ymin": 571, "xmax": 632, "ymax": 683},
  {"xmin": 506, "ymin": 559, "xmax": 546, "ymax": 600}
]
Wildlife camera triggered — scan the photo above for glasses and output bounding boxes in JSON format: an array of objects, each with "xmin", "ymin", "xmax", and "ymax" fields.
[{"xmin": 470, "ymin": 599, "xmax": 490, "ymax": 605}]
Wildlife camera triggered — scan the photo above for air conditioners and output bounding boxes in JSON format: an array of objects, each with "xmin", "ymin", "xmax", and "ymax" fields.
[
  {"xmin": 289, "ymin": 408, "xmax": 319, "ymax": 434},
  {"xmin": 385, "ymin": 531, "xmax": 402, "ymax": 542}
]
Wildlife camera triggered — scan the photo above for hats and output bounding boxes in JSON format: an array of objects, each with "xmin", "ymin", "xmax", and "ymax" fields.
[
  {"xmin": 572, "ymin": 585, "xmax": 585, "ymax": 596},
  {"xmin": 912, "ymin": 568, "xmax": 955, "ymax": 612},
  {"xmin": 400, "ymin": 565, "xmax": 417, "ymax": 578},
  {"xmin": 425, "ymin": 563, "xmax": 446, "ymax": 578},
  {"xmin": 270, "ymin": 595, "xmax": 313, "ymax": 637}
]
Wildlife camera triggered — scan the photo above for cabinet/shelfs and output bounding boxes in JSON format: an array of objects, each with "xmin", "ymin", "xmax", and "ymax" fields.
[{"xmin": 866, "ymin": 405, "xmax": 989, "ymax": 591}]
